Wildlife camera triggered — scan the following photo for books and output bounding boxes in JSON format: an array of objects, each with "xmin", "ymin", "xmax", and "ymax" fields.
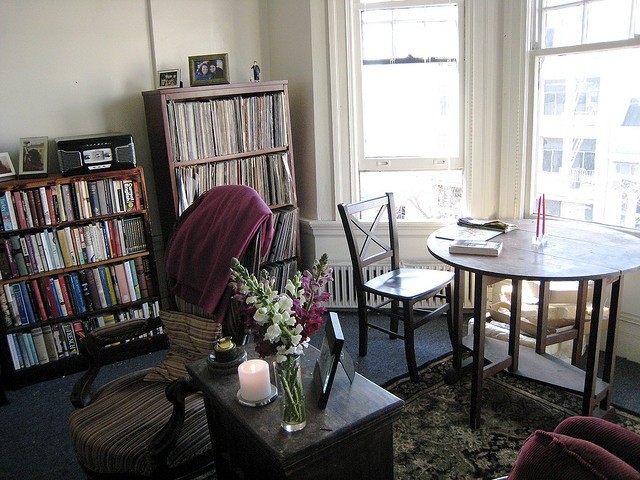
[
  {"xmin": 166, "ymin": 91, "xmax": 288, "ymax": 161},
  {"xmin": 449, "ymin": 238, "xmax": 503, "ymax": 256},
  {"xmin": 261, "ymin": 206, "xmax": 298, "ymax": 264},
  {"xmin": 0, "ymin": 256, "xmax": 155, "ymax": 329},
  {"xmin": 0, "ymin": 216, "xmax": 147, "ymax": 281},
  {"xmin": 259, "ymin": 259, "xmax": 298, "ymax": 296},
  {"xmin": 0, "ymin": 176, "xmax": 141, "ymax": 235},
  {"xmin": 457, "ymin": 216, "xmax": 521, "ymax": 234},
  {"xmin": 174, "ymin": 151, "xmax": 293, "ymax": 217},
  {"xmin": 6, "ymin": 300, "xmax": 166, "ymax": 369}
]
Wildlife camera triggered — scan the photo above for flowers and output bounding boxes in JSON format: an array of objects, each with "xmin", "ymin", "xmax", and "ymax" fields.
[{"xmin": 226, "ymin": 252, "xmax": 334, "ymax": 361}]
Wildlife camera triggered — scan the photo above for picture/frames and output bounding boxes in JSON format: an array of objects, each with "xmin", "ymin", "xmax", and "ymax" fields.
[
  {"xmin": 0, "ymin": 151, "xmax": 14, "ymax": 176},
  {"xmin": 157, "ymin": 68, "xmax": 182, "ymax": 90},
  {"xmin": 312, "ymin": 311, "xmax": 345, "ymax": 409},
  {"xmin": 18, "ymin": 139, "xmax": 49, "ymax": 171},
  {"xmin": 189, "ymin": 54, "xmax": 233, "ymax": 84}
]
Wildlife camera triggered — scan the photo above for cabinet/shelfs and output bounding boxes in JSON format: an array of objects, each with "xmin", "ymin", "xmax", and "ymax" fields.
[
  {"xmin": 143, "ymin": 80, "xmax": 301, "ymax": 308},
  {"xmin": 0, "ymin": 170, "xmax": 171, "ymax": 390}
]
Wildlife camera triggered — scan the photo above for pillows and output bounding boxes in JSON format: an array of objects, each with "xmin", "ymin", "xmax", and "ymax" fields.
[{"xmin": 143, "ymin": 309, "xmax": 222, "ymax": 395}]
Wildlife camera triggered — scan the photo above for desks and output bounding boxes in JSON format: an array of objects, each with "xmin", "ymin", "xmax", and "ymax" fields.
[
  {"xmin": 186, "ymin": 334, "xmax": 406, "ymax": 480},
  {"xmin": 428, "ymin": 219, "xmax": 636, "ymax": 430}
]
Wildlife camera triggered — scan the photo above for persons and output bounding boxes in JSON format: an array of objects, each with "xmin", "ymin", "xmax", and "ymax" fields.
[
  {"xmin": 251, "ymin": 61, "xmax": 261, "ymax": 82},
  {"xmin": 209, "ymin": 63, "xmax": 223, "ymax": 78},
  {"xmin": 196, "ymin": 64, "xmax": 209, "ymax": 79}
]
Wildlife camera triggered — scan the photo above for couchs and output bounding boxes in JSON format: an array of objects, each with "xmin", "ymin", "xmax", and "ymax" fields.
[
  {"xmin": 69, "ymin": 187, "xmax": 272, "ymax": 479},
  {"xmin": 508, "ymin": 417, "xmax": 640, "ymax": 478}
]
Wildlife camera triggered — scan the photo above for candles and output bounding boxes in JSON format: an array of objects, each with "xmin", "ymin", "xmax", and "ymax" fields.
[{"xmin": 238, "ymin": 358, "xmax": 270, "ymax": 400}]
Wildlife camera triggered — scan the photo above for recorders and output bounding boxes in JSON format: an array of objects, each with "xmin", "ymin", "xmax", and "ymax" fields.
[{"xmin": 55, "ymin": 131, "xmax": 137, "ymax": 177}]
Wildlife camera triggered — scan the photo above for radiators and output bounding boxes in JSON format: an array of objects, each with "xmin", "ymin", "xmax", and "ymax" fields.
[{"xmin": 320, "ymin": 262, "xmax": 476, "ymax": 311}]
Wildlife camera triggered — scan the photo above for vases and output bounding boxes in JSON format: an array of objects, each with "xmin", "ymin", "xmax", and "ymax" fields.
[{"xmin": 272, "ymin": 356, "xmax": 306, "ymax": 429}]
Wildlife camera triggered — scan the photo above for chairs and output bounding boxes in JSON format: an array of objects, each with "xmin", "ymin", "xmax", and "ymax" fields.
[{"xmin": 337, "ymin": 194, "xmax": 461, "ymax": 384}]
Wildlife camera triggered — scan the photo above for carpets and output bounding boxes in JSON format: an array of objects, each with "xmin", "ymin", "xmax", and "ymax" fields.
[{"xmin": 375, "ymin": 348, "xmax": 639, "ymax": 478}]
